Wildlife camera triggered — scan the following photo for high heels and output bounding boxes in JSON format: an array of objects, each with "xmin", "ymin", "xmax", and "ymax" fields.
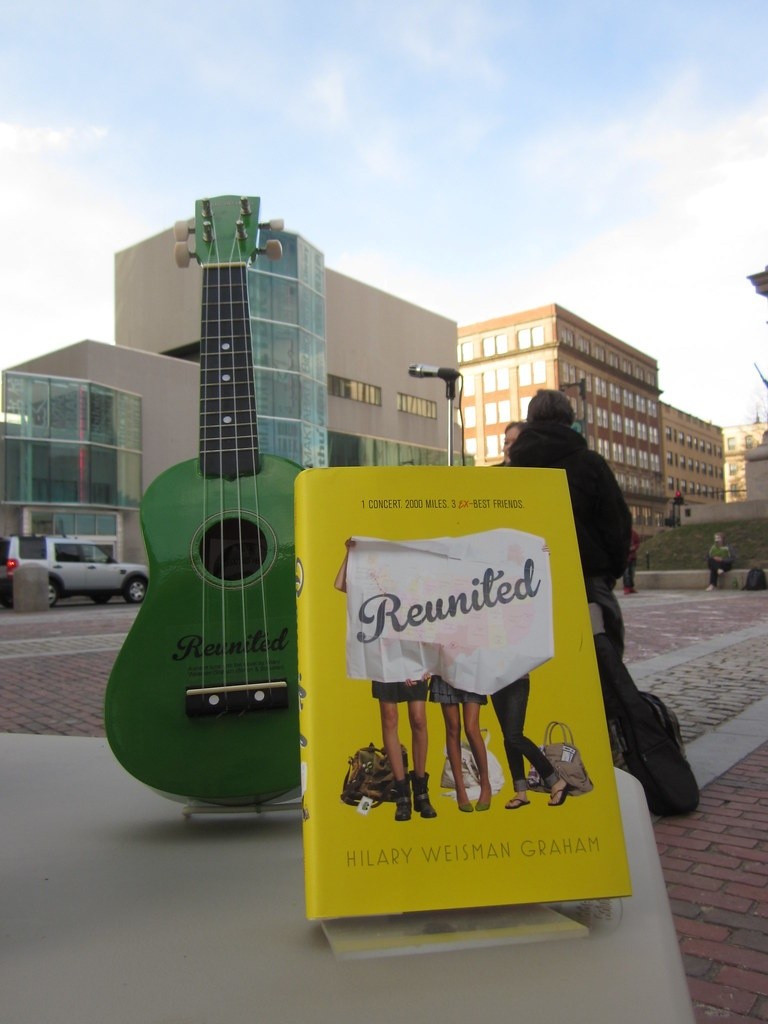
[
  {"xmin": 458, "ymin": 802, "xmax": 474, "ymax": 812},
  {"xmin": 475, "ymin": 803, "xmax": 491, "ymax": 812}
]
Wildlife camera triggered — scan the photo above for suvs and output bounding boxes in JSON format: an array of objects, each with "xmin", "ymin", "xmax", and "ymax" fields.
[{"xmin": 0, "ymin": 533, "xmax": 148, "ymax": 606}]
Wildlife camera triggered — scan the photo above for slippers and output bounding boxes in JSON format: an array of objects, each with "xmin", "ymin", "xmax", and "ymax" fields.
[
  {"xmin": 505, "ymin": 798, "xmax": 530, "ymax": 809},
  {"xmin": 548, "ymin": 783, "xmax": 569, "ymax": 806}
]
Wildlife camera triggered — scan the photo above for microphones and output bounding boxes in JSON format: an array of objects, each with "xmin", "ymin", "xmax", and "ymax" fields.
[{"xmin": 408, "ymin": 364, "xmax": 460, "ymax": 380}]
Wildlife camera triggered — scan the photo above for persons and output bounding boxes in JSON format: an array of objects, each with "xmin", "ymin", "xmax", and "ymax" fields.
[
  {"xmin": 333, "ymin": 536, "xmax": 438, "ymax": 821},
  {"xmin": 624, "ymin": 530, "xmax": 638, "ymax": 595},
  {"xmin": 492, "ymin": 422, "xmax": 530, "ymax": 471},
  {"xmin": 507, "ymin": 388, "xmax": 638, "ymax": 658},
  {"xmin": 426, "ymin": 667, "xmax": 491, "ymax": 814},
  {"xmin": 705, "ymin": 532, "xmax": 735, "ymax": 592},
  {"xmin": 490, "ymin": 672, "xmax": 571, "ymax": 810}
]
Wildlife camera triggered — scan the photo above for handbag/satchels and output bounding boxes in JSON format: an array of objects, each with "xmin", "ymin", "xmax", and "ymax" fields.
[
  {"xmin": 439, "ymin": 728, "xmax": 491, "ymax": 789},
  {"xmin": 527, "ymin": 721, "xmax": 594, "ymax": 798},
  {"xmin": 339, "ymin": 741, "xmax": 409, "ymax": 809}
]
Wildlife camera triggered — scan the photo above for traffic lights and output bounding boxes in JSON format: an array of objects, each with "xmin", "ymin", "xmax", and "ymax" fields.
[{"xmin": 673, "ymin": 491, "xmax": 684, "ymax": 505}]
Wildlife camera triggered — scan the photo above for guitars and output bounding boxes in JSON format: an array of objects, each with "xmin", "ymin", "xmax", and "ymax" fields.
[{"xmin": 105, "ymin": 195, "xmax": 307, "ymax": 807}]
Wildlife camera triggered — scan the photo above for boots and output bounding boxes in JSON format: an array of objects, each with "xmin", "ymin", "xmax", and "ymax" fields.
[
  {"xmin": 408, "ymin": 769, "xmax": 437, "ymax": 818},
  {"xmin": 394, "ymin": 773, "xmax": 412, "ymax": 821}
]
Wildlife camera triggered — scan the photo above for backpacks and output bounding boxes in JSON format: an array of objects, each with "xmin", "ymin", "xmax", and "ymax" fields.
[
  {"xmin": 746, "ymin": 567, "xmax": 767, "ymax": 591},
  {"xmin": 608, "ymin": 691, "xmax": 699, "ymax": 817}
]
